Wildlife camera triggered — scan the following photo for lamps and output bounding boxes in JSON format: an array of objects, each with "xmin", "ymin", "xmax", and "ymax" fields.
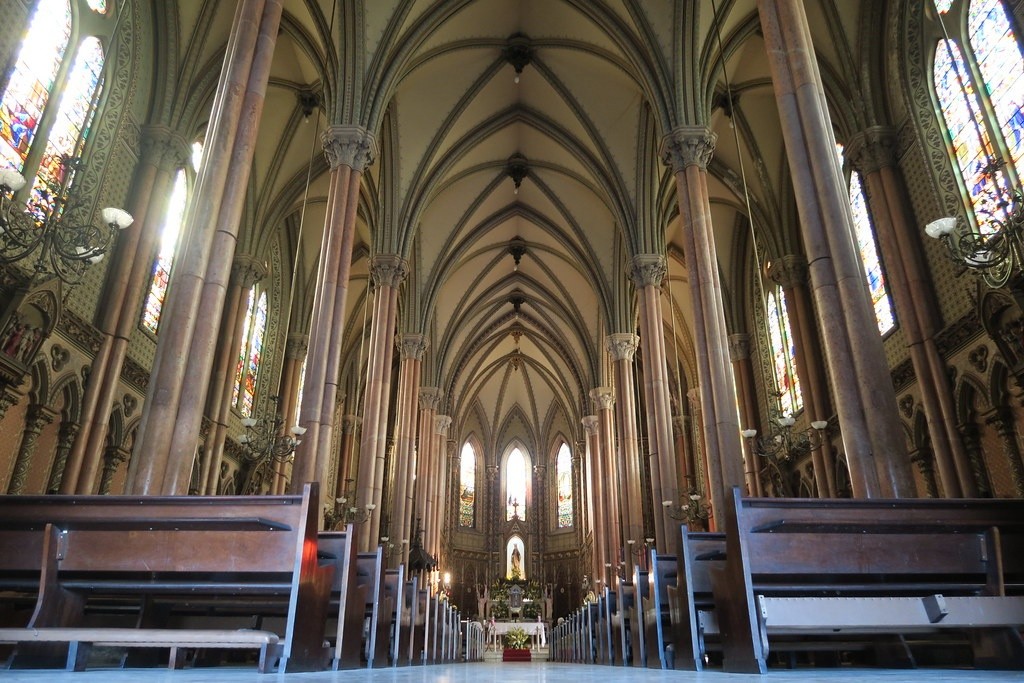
[
  {"xmin": 603, "ymin": 558, "xmax": 627, "ymax": 577},
  {"xmin": 626, "ymin": 281, "xmax": 657, "ymax": 560},
  {"xmin": 0, "ymin": 0, "xmax": 135, "ymax": 286},
  {"xmin": 922, "ymin": 0, "xmax": 1024, "ymax": 291},
  {"xmin": 324, "ymin": 120, "xmax": 387, "ymax": 526},
  {"xmin": 651, "ymin": 116, "xmax": 715, "ymax": 529},
  {"xmin": 236, "ymin": 0, "xmax": 337, "ymax": 480},
  {"xmin": 710, "ymin": 0, "xmax": 830, "ymax": 470},
  {"xmin": 379, "ymin": 283, "xmax": 408, "ymax": 559}
]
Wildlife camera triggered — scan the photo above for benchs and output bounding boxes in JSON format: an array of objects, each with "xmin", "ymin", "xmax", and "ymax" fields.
[
  {"xmin": 0, "ymin": 483, "xmax": 484, "ymax": 674},
  {"xmin": 545, "ymin": 483, "xmax": 1024, "ymax": 675}
]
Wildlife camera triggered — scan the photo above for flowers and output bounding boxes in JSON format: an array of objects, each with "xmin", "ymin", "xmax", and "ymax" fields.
[{"xmin": 489, "ymin": 579, "xmax": 543, "ymax": 650}]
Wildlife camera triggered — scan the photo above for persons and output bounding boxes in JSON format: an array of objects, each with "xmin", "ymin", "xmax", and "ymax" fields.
[
  {"xmin": 5, "ymin": 323, "xmax": 42, "ymax": 363},
  {"xmin": 511, "ymin": 545, "xmax": 521, "ymax": 569},
  {"xmin": 998, "ymin": 323, "xmax": 1024, "ymax": 360}
]
